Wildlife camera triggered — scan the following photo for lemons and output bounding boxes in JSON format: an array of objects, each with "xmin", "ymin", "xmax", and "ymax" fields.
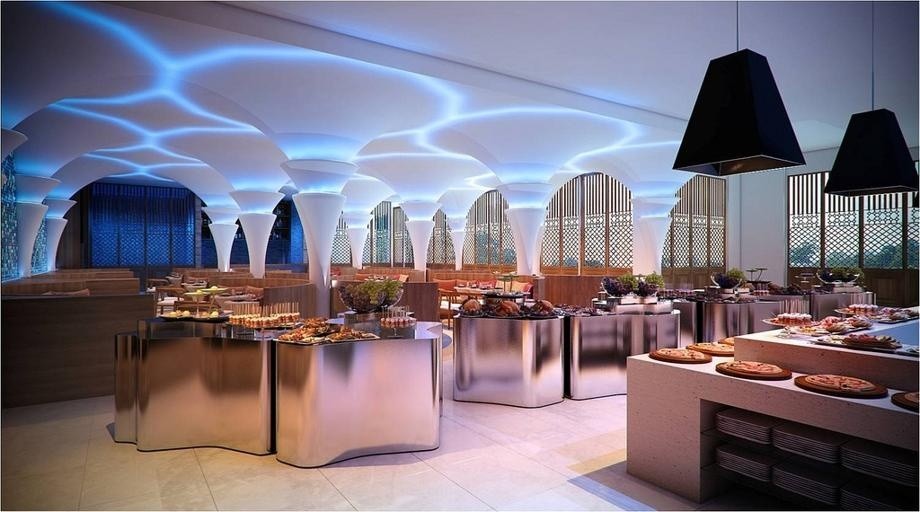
[{"xmin": 168, "ymin": 285, "xmax": 220, "ymax": 318}]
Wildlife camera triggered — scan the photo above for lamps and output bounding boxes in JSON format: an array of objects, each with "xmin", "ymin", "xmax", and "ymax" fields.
[
  {"xmin": 672, "ymin": 0, "xmax": 806, "ymax": 177},
  {"xmin": 824, "ymin": 0, "xmax": 920, "ymax": 197}
]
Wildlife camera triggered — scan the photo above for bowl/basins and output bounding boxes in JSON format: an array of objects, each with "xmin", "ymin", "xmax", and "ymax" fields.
[
  {"xmin": 337, "ymin": 287, "xmax": 387, "ymax": 312},
  {"xmin": 633, "ymin": 280, "xmax": 659, "ymax": 298},
  {"xmin": 711, "ymin": 272, "xmax": 744, "ymax": 289},
  {"xmin": 376, "ymin": 289, "xmax": 404, "ymax": 311},
  {"xmin": 815, "ymin": 271, "xmax": 860, "ymax": 285},
  {"xmin": 600, "ymin": 274, "xmax": 634, "ymax": 298}
]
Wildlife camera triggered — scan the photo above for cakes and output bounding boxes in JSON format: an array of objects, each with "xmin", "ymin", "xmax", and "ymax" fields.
[
  {"xmin": 228, "ymin": 311, "xmax": 301, "ymax": 328},
  {"xmin": 381, "ymin": 315, "xmax": 418, "ymax": 328},
  {"xmin": 849, "ymin": 302, "xmax": 879, "ymax": 313},
  {"xmin": 776, "ymin": 312, "xmax": 812, "ymax": 326}
]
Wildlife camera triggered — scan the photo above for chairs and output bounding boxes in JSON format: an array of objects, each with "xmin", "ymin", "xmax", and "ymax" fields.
[
  {"xmin": 355, "ymin": 272, "xmax": 409, "ymax": 282},
  {"xmin": 433, "ymin": 273, "xmax": 533, "ymax": 330},
  {"xmin": 143, "ymin": 271, "xmax": 267, "ymax": 315}
]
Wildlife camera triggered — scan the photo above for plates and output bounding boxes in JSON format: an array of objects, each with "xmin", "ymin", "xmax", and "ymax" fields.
[
  {"xmin": 712, "ymin": 408, "xmax": 918, "ymax": 511},
  {"xmin": 457, "ymin": 305, "xmax": 603, "ymax": 320},
  {"xmin": 759, "ymin": 310, "xmax": 920, "ymax": 336},
  {"xmin": 808, "ymin": 335, "xmax": 919, "ymax": 356},
  {"xmin": 272, "ymin": 324, "xmax": 381, "ymax": 346},
  {"xmin": 160, "ymin": 309, "xmax": 230, "ymax": 322}
]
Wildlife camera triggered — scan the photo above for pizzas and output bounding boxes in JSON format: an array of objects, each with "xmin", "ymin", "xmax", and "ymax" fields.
[
  {"xmin": 904, "ymin": 390, "xmax": 919, "ymax": 404},
  {"xmin": 656, "ymin": 347, "xmax": 704, "ymax": 360},
  {"xmin": 805, "ymin": 373, "xmax": 876, "ymax": 393},
  {"xmin": 691, "ymin": 342, "xmax": 735, "ymax": 352},
  {"xmin": 718, "ymin": 359, "xmax": 783, "ymax": 375},
  {"xmin": 724, "ymin": 336, "xmax": 735, "ymax": 344}
]
